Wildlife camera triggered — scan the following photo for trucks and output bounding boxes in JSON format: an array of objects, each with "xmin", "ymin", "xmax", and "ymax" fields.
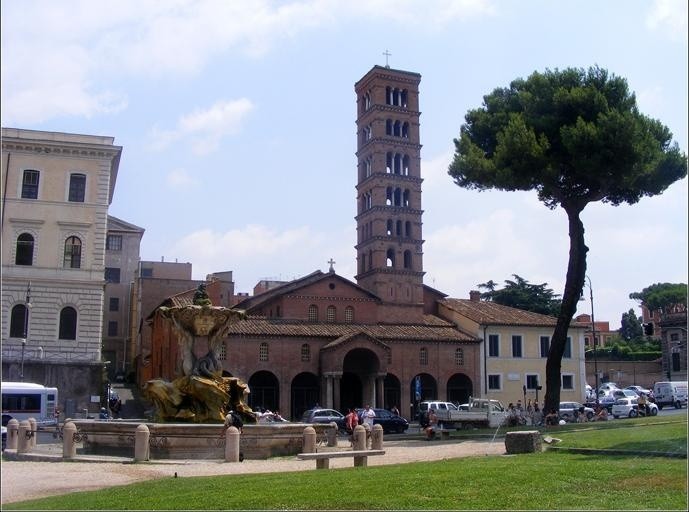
[{"xmin": 435, "ymin": 396, "xmax": 510, "ymax": 428}]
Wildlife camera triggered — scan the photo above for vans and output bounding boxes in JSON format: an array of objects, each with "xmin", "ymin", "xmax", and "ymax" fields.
[
  {"xmin": 654, "ymin": 381, "xmax": 688, "ymax": 410},
  {"xmin": 418, "ymin": 402, "xmax": 457, "ymax": 425}
]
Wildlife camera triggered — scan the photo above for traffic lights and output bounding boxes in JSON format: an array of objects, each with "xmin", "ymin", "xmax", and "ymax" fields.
[{"xmin": 534, "ymin": 386, "xmax": 542, "ymax": 390}]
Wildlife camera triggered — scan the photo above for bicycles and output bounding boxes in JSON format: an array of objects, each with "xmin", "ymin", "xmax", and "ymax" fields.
[
  {"xmin": 118, "ymin": 435, "xmax": 169, "ymax": 447},
  {"xmin": 54, "ymin": 425, "xmax": 88, "ymax": 442}
]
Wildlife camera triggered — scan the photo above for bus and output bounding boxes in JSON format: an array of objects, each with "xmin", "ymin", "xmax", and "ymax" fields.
[{"xmin": 1, "ymin": 381, "xmax": 58, "ymax": 438}]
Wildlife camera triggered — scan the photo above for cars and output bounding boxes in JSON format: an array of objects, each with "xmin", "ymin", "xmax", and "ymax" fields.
[
  {"xmin": 303, "ymin": 409, "xmax": 345, "ymax": 428},
  {"xmin": 559, "ymin": 402, "xmax": 596, "ymax": 422},
  {"xmin": 586, "ymin": 382, "xmax": 659, "ymax": 419},
  {"xmin": 356, "ymin": 408, "xmax": 409, "ymax": 433}
]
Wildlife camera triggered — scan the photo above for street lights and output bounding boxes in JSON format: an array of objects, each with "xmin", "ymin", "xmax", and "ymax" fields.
[
  {"xmin": 20, "ymin": 280, "xmax": 33, "ymax": 380},
  {"xmin": 579, "ymin": 275, "xmax": 599, "ymax": 406}
]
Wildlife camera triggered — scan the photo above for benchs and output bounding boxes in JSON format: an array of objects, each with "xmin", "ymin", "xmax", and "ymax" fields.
[
  {"xmin": 297, "ymin": 450, "xmax": 385, "ymax": 469},
  {"xmin": 432, "ymin": 428, "xmax": 456, "ymax": 439}
]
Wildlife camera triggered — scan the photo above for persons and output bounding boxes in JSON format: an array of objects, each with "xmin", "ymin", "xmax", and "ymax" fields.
[
  {"xmin": 99, "ymin": 407, "xmax": 108, "ymax": 419},
  {"xmin": 346, "ymin": 408, "xmax": 359, "ymax": 434},
  {"xmin": 637, "ymin": 392, "xmax": 646, "ymax": 418},
  {"xmin": 589, "ymin": 406, "xmax": 608, "ymax": 422},
  {"xmin": 647, "ymin": 389, "xmax": 655, "ymax": 403},
  {"xmin": 361, "ymin": 405, "xmax": 376, "ymax": 449},
  {"xmin": 391, "ymin": 406, "xmax": 400, "ymax": 417},
  {"xmin": 221, "ymin": 405, "xmax": 244, "ymax": 462},
  {"xmin": 425, "ymin": 409, "xmax": 438, "ymax": 441},
  {"xmin": 251, "ymin": 406, "xmax": 289, "ymax": 424},
  {"xmin": 505, "ymin": 399, "xmax": 559, "ymax": 428}
]
